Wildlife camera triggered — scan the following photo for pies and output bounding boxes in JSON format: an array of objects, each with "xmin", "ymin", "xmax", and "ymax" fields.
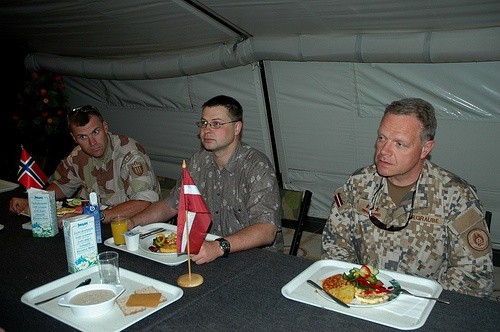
[{"xmin": 329, "ymin": 284, "xmax": 355, "ymax": 303}]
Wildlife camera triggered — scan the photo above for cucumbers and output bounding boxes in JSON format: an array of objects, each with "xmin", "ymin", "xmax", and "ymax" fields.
[{"xmin": 67, "ymin": 200, "xmax": 82, "ymax": 206}]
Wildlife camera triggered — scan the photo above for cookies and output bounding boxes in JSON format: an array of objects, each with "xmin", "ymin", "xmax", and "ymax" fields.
[{"xmin": 115, "ymin": 286, "xmax": 167, "ymax": 316}]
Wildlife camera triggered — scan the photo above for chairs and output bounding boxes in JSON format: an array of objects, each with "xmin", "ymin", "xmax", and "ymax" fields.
[{"xmin": 279, "ymin": 189, "xmax": 312, "ymax": 258}]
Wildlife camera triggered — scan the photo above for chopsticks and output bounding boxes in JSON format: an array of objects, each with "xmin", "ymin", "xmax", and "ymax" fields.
[{"xmin": 141, "ymin": 228, "xmax": 165, "ymax": 240}]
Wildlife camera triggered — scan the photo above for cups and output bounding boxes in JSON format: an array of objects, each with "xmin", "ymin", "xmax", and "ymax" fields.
[
  {"xmin": 124, "ymin": 232, "xmax": 140, "ymax": 252},
  {"xmin": 96, "ymin": 251, "xmax": 121, "ymax": 285},
  {"xmin": 110, "ymin": 214, "xmax": 128, "ymax": 246}
]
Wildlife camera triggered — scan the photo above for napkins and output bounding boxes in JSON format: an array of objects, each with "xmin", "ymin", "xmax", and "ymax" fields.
[
  {"xmin": 383, "ymin": 289, "xmax": 430, "ymax": 319},
  {"xmin": 128, "ymin": 226, "xmax": 149, "ymax": 234}
]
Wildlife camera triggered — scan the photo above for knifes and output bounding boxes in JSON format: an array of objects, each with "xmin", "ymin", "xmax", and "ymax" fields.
[{"xmin": 306, "ymin": 280, "xmax": 350, "ymax": 308}]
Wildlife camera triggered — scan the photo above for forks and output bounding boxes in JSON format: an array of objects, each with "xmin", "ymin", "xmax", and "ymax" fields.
[{"xmin": 400, "ymin": 289, "xmax": 450, "ymax": 306}]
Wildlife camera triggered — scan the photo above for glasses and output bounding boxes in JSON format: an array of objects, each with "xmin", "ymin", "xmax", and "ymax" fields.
[
  {"xmin": 367, "ymin": 184, "xmax": 417, "ymax": 232},
  {"xmin": 66, "ymin": 105, "xmax": 101, "ymax": 120},
  {"xmin": 195, "ymin": 120, "xmax": 238, "ymax": 129}
]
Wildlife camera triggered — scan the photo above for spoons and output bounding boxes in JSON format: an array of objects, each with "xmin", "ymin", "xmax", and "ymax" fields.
[{"xmin": 34, "ymin": 278, "xmax": 91, "ymax": 305}]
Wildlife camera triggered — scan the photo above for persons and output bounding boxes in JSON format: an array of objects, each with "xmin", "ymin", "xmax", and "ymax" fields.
[
  {"xmin": 126, "ymin": 95, "xmax": 285, "ymax": 265},
  {"xmin": 321, "ymin": 98, "xmax": 494, "ymax": 298},
  {"xmin": 9, "ymin": 105, "xmax": 161, "ymax": 224}
]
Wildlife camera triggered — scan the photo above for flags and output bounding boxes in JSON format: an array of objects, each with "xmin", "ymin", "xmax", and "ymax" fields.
[
  {"xmin": 18, "ymin": 149, "xmax": 48, "ymax": 191},
  {"xmin": 176, "ymin": 168, "xmax": 211, "ymax": 255}
]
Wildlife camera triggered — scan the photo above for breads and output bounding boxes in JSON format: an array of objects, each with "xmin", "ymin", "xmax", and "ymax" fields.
[{"xmin": 160, "ymin": 247, "xmax": 177, "ymax": 252}]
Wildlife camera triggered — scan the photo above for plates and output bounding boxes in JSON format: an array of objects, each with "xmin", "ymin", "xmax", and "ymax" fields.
[
  {"xmin": 139, "ymin": 229, "xmax": 178, "ymax": 255},
  {"xmin": 316, "ymin": 268, "xmax": 401, "ymax": 308}
]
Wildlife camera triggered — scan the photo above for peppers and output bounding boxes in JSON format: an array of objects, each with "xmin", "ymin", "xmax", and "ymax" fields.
[
  {"xmin": 342, "ymin": 265, "xmax": 392, "ymax": 293},
  {"xmin": 154, "ymin": 233, "xmax": 171, "ymax": 247}
]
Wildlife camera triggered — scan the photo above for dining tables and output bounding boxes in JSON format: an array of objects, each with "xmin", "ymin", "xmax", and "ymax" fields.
[{"xmin": 0, "ymin": 177, "xmax": 500, "ymax": 332}]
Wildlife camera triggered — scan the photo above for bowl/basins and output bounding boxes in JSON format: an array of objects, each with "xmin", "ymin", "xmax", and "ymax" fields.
[{"xmin": 58, "ymin": 284, "xmax": 125, "ymax": 322}]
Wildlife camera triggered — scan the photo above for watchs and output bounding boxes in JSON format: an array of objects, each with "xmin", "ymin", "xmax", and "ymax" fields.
[{"xmin": 215, "ymin": 238, "xmax": 230, "ymax": 256}]
